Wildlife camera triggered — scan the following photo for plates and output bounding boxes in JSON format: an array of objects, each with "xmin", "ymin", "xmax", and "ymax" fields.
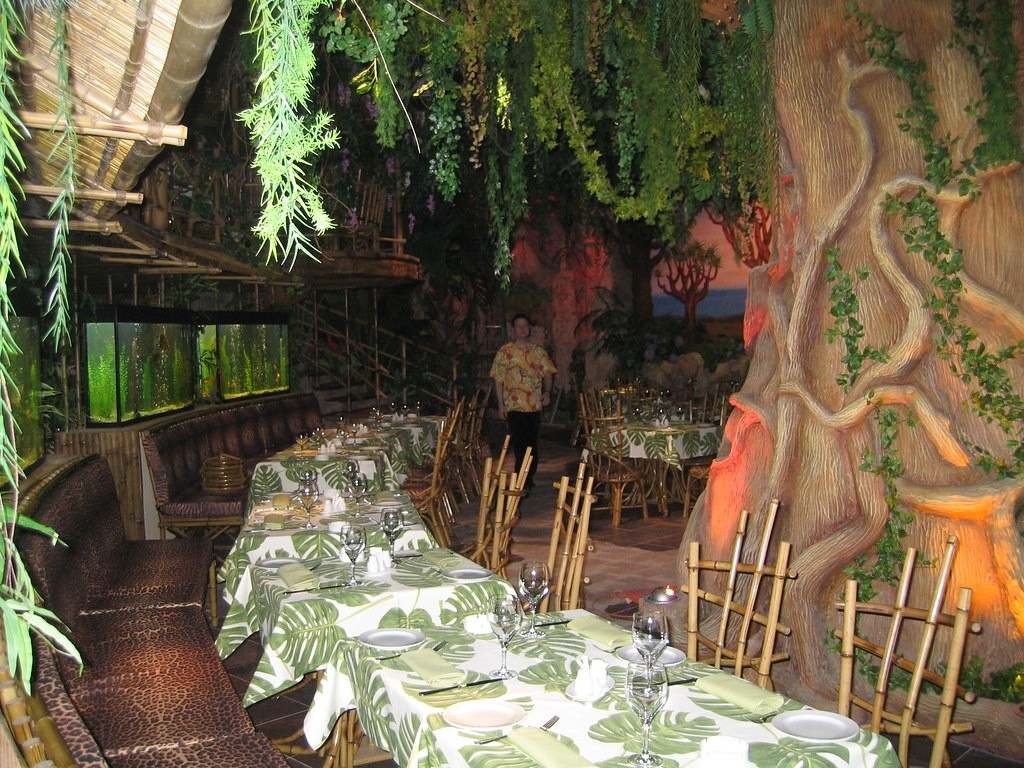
[
  {"xmin": 371, "ymin": 499, "xmax": 406, "ymax": 510},
  {"xmin": 655, "ymin": 428, "xmax": 679, "ymax": 433},
  {"xmin": 345, "ymin": 455, "xmax": 371, "ymax": 460},
  {"xmin": 256, "ymin": 557, "xmax": 304, "ymax": 570},
  {"xmin": 442, "ymin": 569, "xmax": 494, "ymax": 582},
  {"xmin": 360, "ymin": 446, "xmax": 384, "ymax": 452},
  {"xmin": 443, "ymin": 699, "xmax": 527, "ymax": 729},
  {"xmin": 773, "ymin": 710, "xmax": 859, "ymax": 743},
  {"xmin": 611, "ymin": 642, "xmax": 688, "ymax": 670},
  {"xmin": 359, "ymin": 628, "xmax": 425, "ymax": 650}
]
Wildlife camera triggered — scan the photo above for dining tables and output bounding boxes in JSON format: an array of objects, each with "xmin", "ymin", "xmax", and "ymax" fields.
[{"xmin": 212, "ymin": 415, "xmax": 903, "ymax": 768}]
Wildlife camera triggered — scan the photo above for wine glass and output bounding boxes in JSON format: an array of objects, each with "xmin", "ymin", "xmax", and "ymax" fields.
[
  {"xmin": 612, "ymin": 377, "xmax": 689, "ymax": 428},
  {"xmin": 489, "ymin": 560, "xmax": 668, "ymax": 765},
  {"xmin": 294, "ymin": 400, "xmax": 423, "ymax": 588}
]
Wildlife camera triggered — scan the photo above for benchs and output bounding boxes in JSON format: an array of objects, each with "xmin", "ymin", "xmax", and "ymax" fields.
[
  {"xmin": 137, "ymin": 389, "xmax": 325, "ymax": 542},
  {"xmin": 0, "ymin": 451, "xmax": 296, "ymax": 768}
]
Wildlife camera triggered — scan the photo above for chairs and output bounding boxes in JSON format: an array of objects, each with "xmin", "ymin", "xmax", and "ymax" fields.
[
  {"xmin": 579, "ymin": 376, "xmax": 738, "ymax": 526},
  {"xmin": 832, "ymin": 534, "xmax": 979, "ymax": 768},
  {"xmin": 630, "ymin": 499, "xmax": 796, "ymax": 696},
  {"xmin": 404, "ymin": 383, "xmax": 534, "ymax": 583},
  {"xmin": 522, "ymin": 460, "xmax": 594, "ymax": 613}
]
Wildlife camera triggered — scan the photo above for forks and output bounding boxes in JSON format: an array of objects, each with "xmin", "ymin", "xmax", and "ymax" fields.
[
  {"xmin": 472, "ymin": 716, "xmax": 559, "ymax": 746},
  {"xmin": 744, "ymin": 707, "xmax": 812, "ymax": 724},
  {"xmin": 376, "ymin": 641, "xmax": 446, "ymax": 660},
  {"xmin": 265, "ymin": 563, "xmax": 321, "ymax": 578}
]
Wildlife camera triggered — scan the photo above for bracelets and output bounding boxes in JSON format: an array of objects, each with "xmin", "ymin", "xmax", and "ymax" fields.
[{"xmin": 544, "ymin": 390, "xmax": 551, "ymax": 393}]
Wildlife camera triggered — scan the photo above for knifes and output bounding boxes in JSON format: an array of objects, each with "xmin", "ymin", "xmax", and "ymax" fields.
[
  {"xmin": 283, "ymin": 584, "xmax": 349, "ymax": 595},
  {"xmin": 419, "ymin": 678, "xmax": 508, "ymax": 697}
]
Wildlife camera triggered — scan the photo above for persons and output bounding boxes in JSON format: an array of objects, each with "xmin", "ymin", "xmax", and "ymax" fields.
[{"xmin": 490, "ymin": 313, "xmax": 558, "ymax": 489}]
